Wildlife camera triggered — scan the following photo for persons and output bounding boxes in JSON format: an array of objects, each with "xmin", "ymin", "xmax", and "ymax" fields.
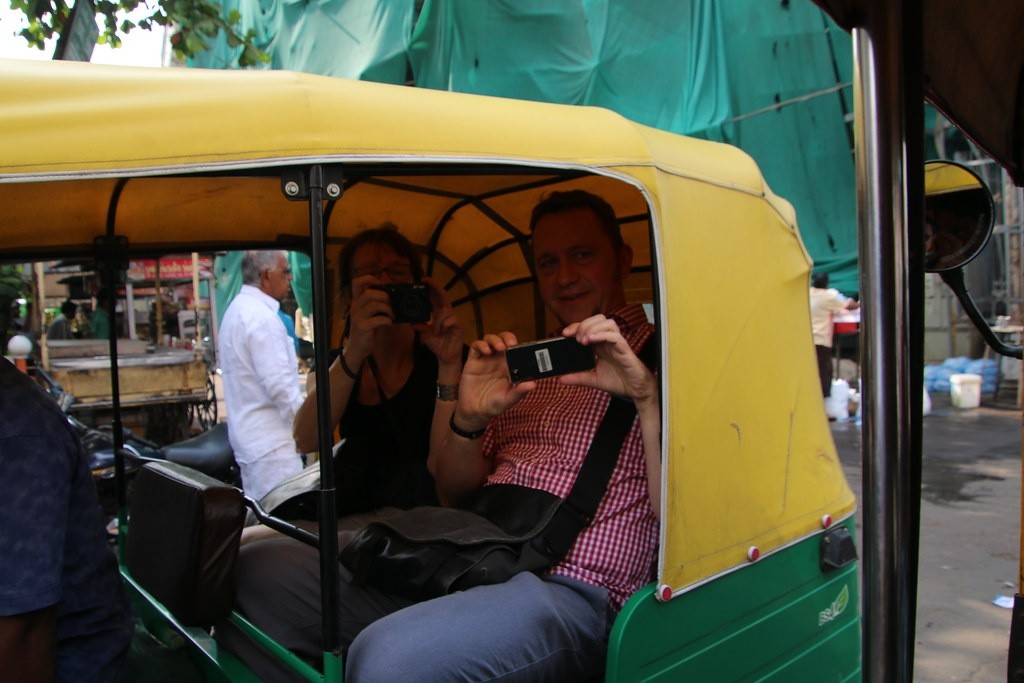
[
  {"xmin": 87, "ymin": 289, "xmax": 117, "ymax": 338},
  {"xmin": 46, "ymin": 302, "xmax": 77, "ymax": 340},
  {"xmin": 207, "ymin": 190, "xmax": 661, "ymax": 683},
  {"xmin": 218, "ymin": 250, "xmax": 307, "ymax": 524},
  {"xmin": 0, "ymin": 354, "xmax": 134, "ymax": 683},
  {"xmin": 925, "ymin": 223, "xmax": 962, "ymax": 269},
  {"xmin": 148, "ymin": 296, "xmax": 188, "ymax": 346},
  {"xmin": 240, "ymin": 221, "xmax": 471, "ymax": 551},
  {"xmin": 810, "ymin": 273, "xmax": 863, "ymax": 419},
  {"xmin": 11, "ymin": 301, "xmax": 32, "ymax": 336}
]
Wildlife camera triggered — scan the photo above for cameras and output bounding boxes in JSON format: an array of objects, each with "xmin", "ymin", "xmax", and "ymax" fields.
[{"xmin": 368, "ymin": 281, "xmax": 432, "ymax": 323}]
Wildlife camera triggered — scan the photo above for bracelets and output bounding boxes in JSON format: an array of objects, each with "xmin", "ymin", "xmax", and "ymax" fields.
[
  {"xmin": 340, "ymin": 350, "xmax": 357, "ymax": 379},
  {"xmin": 450, "ymin": 410, "xmax": 486, "ymax": 439},
  {"xmin": 436, "ymin": 382, "xmax": 458, "ymax": 401}
]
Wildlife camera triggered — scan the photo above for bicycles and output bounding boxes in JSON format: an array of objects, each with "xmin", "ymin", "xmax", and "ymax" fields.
[{"xmin": 185, "ymin": 336, "xmax": 218, "ymax": 431}]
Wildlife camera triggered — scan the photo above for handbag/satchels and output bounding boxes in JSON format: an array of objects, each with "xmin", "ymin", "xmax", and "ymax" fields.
[{"xmin": 345, "ymin": 479, "xmax": 567, "ymax": 608}]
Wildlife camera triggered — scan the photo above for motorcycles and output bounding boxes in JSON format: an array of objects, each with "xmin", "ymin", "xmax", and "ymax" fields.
[{"xmin": 35, "ymin": 365, "xmax": 308, "ymax": 554}]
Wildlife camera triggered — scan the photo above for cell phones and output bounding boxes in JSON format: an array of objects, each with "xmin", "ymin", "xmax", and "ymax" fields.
[{"xmin": 505, "ymin": 335, "xmax": 598, "ymax": 384}]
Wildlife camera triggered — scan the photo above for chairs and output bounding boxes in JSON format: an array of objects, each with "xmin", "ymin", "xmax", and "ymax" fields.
[{"xmin": 117, "ymin": 458, "xmax": 243, "ymax": 683}]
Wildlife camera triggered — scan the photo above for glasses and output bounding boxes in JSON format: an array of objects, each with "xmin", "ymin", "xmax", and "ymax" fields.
[{"xmin": 353, "ymin": 263, "xmax": 411, "ymax": 284}]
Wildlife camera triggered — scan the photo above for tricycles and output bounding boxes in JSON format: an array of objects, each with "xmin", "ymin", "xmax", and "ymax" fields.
[{"xmin": 0, "ymin": 60, "xmax": 864, "ymax": 683}]
